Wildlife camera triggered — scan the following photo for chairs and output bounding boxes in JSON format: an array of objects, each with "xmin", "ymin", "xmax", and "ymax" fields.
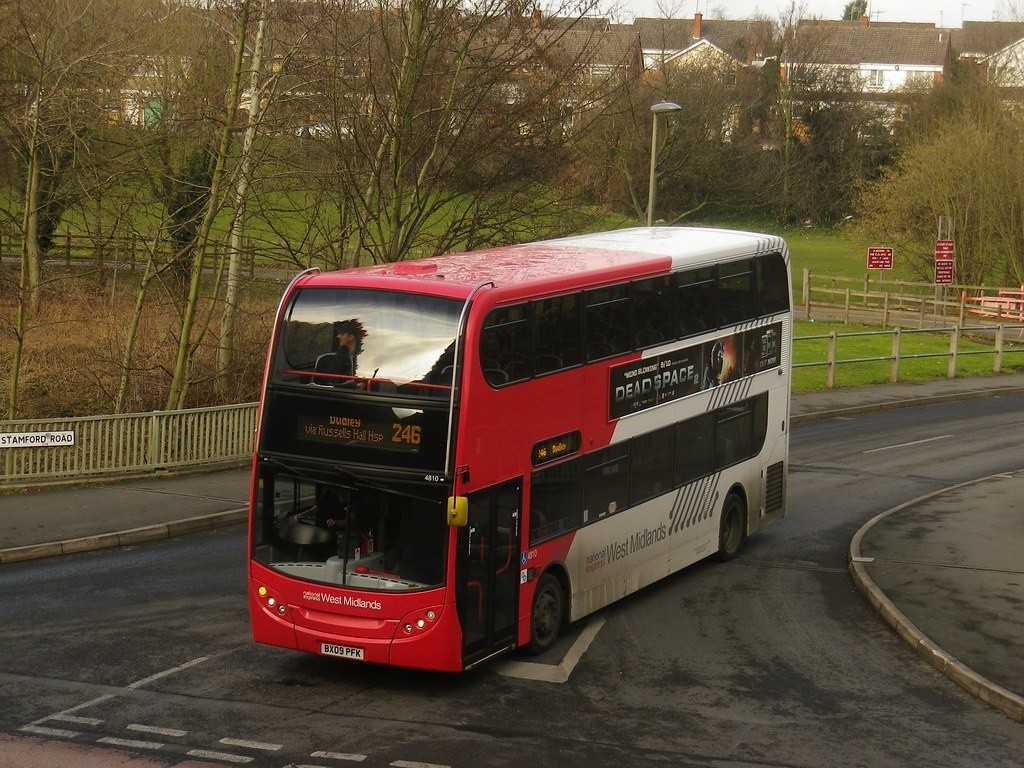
[{"xmin": 311, "ymin": 284, "xmax": 764, "ymax": 393}]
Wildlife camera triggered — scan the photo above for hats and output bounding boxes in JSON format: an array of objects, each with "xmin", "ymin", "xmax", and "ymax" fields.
[{"xmin": 335, "ymin": 323, "xmax": 358, "ymax": 336}]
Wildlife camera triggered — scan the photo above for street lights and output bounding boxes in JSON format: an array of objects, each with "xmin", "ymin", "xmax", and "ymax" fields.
[{"xmin": 646, "ymin": 102, "xmax": 682, "ymax": 228}]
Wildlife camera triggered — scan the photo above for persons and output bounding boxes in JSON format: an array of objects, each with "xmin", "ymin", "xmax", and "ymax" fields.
[
  {"xmin": 293, "ymin": 491, "xmax": 354, "ymax": 528},
  {"xmin": 332, "ymin": 326, "xmax": 371, "ymax": 390}
]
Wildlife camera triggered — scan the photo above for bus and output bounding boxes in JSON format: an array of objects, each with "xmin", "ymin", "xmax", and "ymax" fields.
[{"xmin": 248, "ymin": 227, "xmax": 794, "ymax": 673}]
